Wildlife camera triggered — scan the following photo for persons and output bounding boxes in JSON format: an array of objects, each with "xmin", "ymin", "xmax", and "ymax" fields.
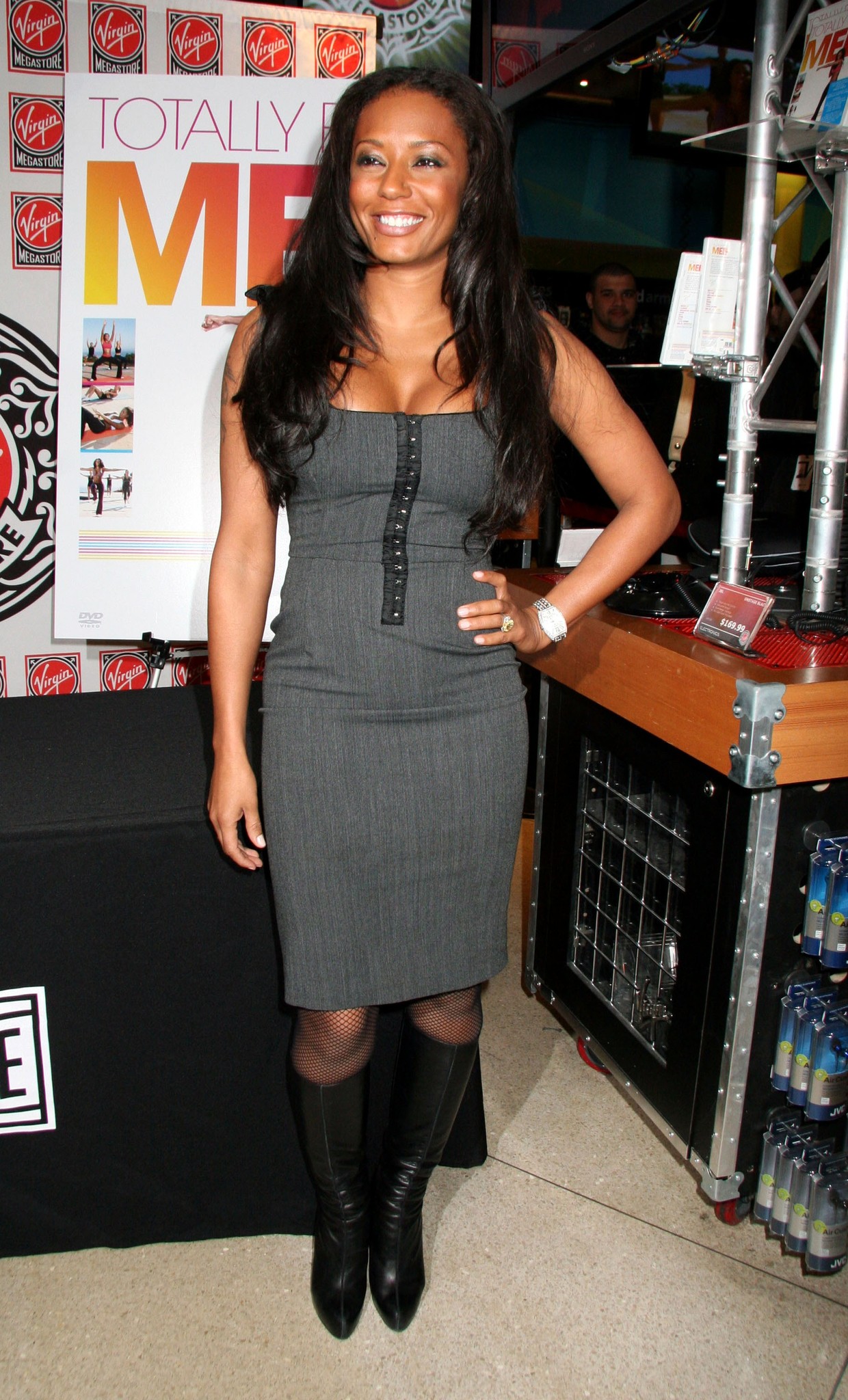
[
  {"xmin": 80, "ymin": 320, "xmax": 133, "ymax": 515},
  {"xmin": 575, "ymin": 265, "xmax": 827, "ymax": 515},
  {"xmin": 204, "ymin": 63, "xmax": 682, "ymax": 1340}
]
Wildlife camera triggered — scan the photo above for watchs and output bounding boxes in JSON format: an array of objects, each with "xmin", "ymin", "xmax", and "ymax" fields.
[{"xmin": 533, "ymin": 597, "xmax": 567, "ymax": 644}]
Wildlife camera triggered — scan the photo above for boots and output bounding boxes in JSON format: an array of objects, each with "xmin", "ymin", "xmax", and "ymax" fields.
[
  {"xmin": 285, "ymin": 1055, "xmax": 371, "ymax": 1338},
  {"xmin": 368, "ymin": 1018, "xmax": 480, "ymax": 1331}
]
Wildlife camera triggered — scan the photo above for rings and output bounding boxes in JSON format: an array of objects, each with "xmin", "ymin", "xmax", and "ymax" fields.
[{"xmin": 499, "ymin": 613, "xmax": 514, "ymax": 633}]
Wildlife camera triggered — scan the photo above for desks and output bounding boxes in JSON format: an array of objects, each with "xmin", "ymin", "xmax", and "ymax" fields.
[{"xmin": 0, "ymin": 681, "xmax": 487, "ymax": 1262}]
